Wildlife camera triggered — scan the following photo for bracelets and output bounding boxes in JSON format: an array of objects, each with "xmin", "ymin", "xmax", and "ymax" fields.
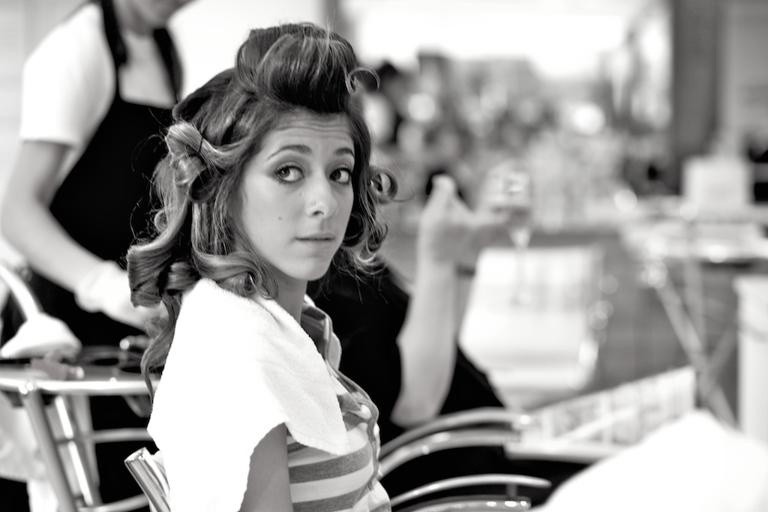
[{"xmin": 457, "ymin": 263, "xmax": 475, "ymax": 277}]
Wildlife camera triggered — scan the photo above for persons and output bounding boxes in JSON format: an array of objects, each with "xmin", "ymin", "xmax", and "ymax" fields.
[
  {"xmin": 0, "ymin": 1, "xmax": 198, "ymax": 512},
  {"xmin": 125, "ymin": 23, "xmax": 390, "ymax": 512},
  {"xmin": 306, "ymin": 158, "xmax": 593, "ymax": 508}
]
